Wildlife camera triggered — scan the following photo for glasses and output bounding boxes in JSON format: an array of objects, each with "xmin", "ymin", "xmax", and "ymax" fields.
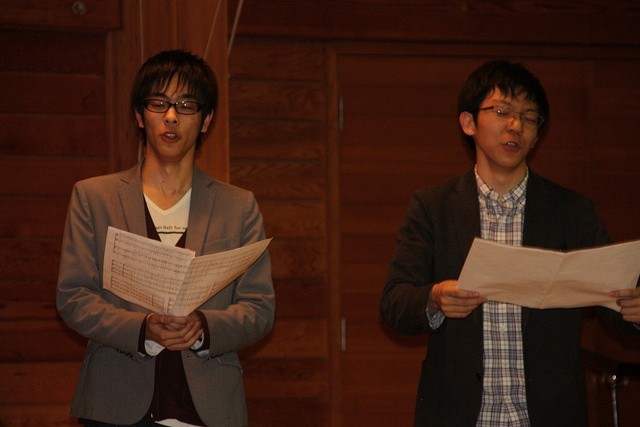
[
  {"xmin": 143, "ymin": 98, "xmax": 205, "ymax": 115},
  {"xmin": 479, "ymin": 104, "xmax": 545, "ymax": 127}
]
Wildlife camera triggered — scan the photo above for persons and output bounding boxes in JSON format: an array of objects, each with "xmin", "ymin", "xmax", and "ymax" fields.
[
  {"xmin": 57, "ymin": 49, "xmax": 277, "ymax": 427},
  {"xmin": 379, "ymin": 59, "xmax": 640, "ymax": 426}
]
